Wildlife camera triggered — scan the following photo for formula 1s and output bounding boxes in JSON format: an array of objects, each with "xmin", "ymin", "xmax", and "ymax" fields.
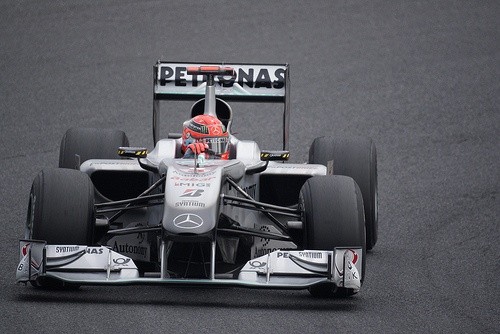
[{"xmin": 14, "ymin": 58, "xmax": 381, "ymax": 302}]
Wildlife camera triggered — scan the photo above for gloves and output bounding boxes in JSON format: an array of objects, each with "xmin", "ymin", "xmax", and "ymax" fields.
[{"xmin": 185, "ymin": 143, "xmax": 209, "ymax": 155}]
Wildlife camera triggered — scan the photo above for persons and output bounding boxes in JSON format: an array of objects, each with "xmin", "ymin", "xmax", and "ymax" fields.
[{"xmin": 181, "ymin": 115, "xmax": 231, "ymax": 160}]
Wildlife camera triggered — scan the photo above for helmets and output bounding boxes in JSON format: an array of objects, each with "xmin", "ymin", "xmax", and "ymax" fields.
[{"xmin": 180, "ymin": 115, "xmax": 231, "ymax": 159}]
